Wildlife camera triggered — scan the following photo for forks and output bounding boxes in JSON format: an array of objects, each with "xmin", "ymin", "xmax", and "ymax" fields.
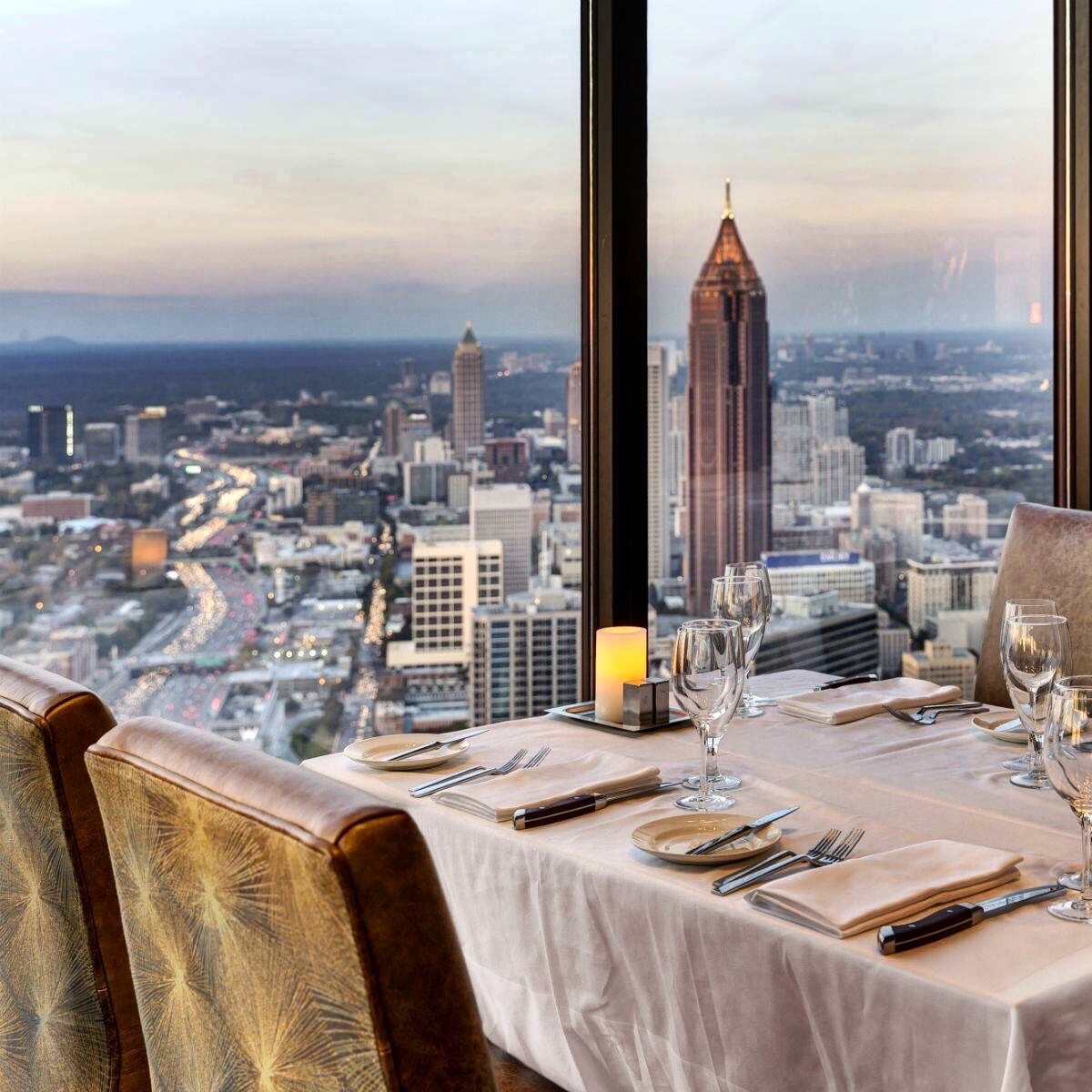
[
  {"xmin": 410, "ymin": 746, "xmax": 550, "ymax": 798},
  {"xmin": 712, "ymin": 828, "xmax": 842, "ymax": 886},
  {"xmin": 880, "ymin": 702, "xmax": 982, "ymax": 721},
  {"xmin": 408, "ymin": 748, "xmax": 529, "ymax": 792},
  {"xmin": 711, "ymin": 827, "xmax": 865, "ymax": 896},
  {"xmin": 894, "ymin": 707, "xmax": 990, "ymax": 725}
]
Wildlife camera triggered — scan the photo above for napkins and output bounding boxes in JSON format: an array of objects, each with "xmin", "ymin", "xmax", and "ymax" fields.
[
  {"xmin": 775, "ymin": 676, "xmax": 961, "ymax": 724},
  {"xmin": 431, "ymin": 750, "xmax": 661, "ymax": 822},
  {"xmin": 742, "ymin": 839, "xmax": 1024, "ymax": 938}
]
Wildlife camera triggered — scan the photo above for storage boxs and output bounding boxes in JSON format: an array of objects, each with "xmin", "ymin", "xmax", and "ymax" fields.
[{"xmin": 623, "ymin": 677, "xmax": 670, "ymax": 727}]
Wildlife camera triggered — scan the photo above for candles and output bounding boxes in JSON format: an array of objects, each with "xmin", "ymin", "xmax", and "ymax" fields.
[{"xmin": 595, "ymin": 625, "xmax": 646, "ymax": 723}]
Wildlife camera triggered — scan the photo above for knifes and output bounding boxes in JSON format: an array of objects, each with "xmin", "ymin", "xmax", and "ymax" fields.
[
  {"xmin": 512, "ymin": 782, "xmax": 683, "ymax": 830},
  {"xmin": 381, "ymin": 729, "xmax": 490, "ymax": 761},
  {"xmin": 685, "ymin": 807, "xmax": 799, "ymax": 855},
  {"xmin": 877, "ymin": 885, "xmax": 1066, "ymax": 956},
  {"xmin": 755, "ymin": 674, "xmax": 878, "ymax": 702},
  {"xmin": 994, "ymin": 716, "xmax": 1022, "ymax": 732}
]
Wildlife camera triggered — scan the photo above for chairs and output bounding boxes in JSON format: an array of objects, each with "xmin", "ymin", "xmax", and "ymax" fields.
[
  {"xmin": 0, "ymin": 653, "xmax": 558, "ymax": 1091},
  {"xmin": 974, "ymin": 500, "xmax": 1092, "ymax": 710}
]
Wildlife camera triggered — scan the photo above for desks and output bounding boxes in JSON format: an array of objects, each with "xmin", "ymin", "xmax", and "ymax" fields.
[{"xmin": 298, "ymin": 669, "xmax": 1092, "ymax": 1091}]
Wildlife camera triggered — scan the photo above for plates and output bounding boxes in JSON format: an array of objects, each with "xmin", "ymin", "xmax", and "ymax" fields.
[
  {"xmin": 343, "ymin": 733, "xmax": 470, "ymax": 771},
  {"xmin": 631, "ymin": 813, "xmax": 782, "ymax": 865},
  {"xmin": 971, "ymin": 710, "xmax": 1088, "ymax": 744}
]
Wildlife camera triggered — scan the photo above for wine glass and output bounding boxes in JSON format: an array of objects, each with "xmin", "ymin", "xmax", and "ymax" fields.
[
  {"xmin": 998, "ymin": 598, "xmax": 1092, "ymax": 923},
  {"xmin": 671, "ymin": 619, "xmax": 745, "ymax": 812},
  {"xmin": 710, "ymin": 562, "xmax": 772, "ymax": 720}
]
